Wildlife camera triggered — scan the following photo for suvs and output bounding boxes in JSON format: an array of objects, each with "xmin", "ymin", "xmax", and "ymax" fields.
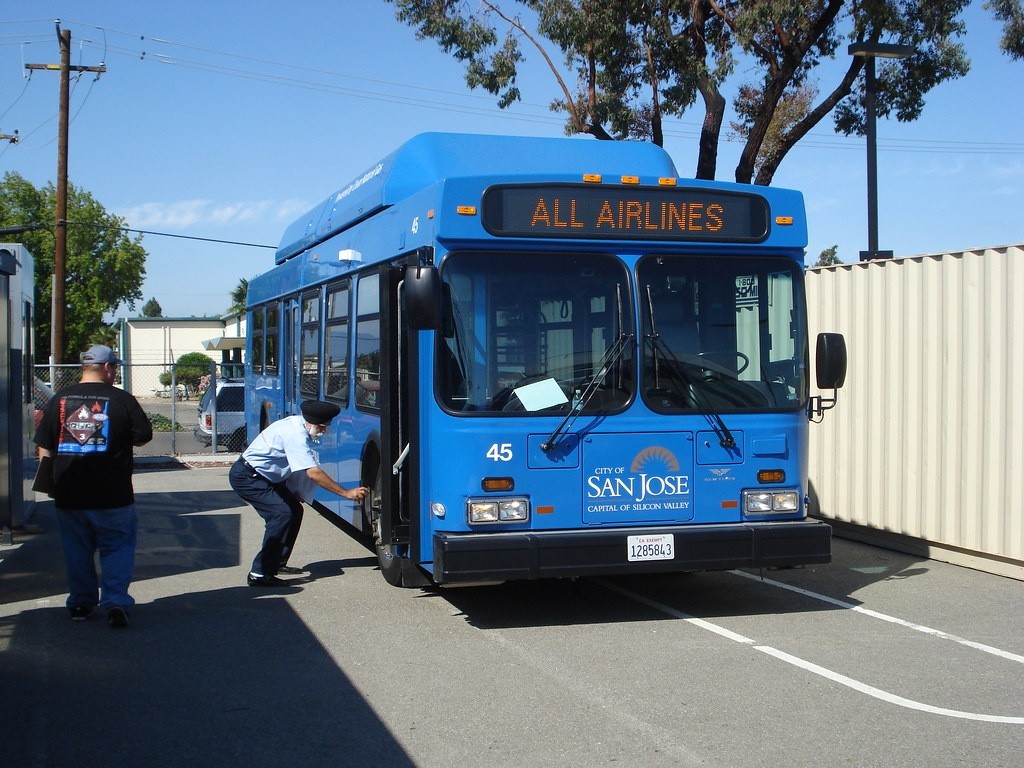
[{"xmin": 194, "ymin": 376, "xmax": 250, "ymax": 454}]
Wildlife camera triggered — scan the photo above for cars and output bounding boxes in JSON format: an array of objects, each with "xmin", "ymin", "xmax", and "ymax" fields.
[{"xmin": 22, "ymin": 375, "xmax": 56, "ymax": 430}]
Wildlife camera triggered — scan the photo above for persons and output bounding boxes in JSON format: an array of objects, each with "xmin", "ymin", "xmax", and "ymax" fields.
[
  {"xmin": 229, "ymin": 400, "xmax": 370, "ymax": 586},
  {"xmin": 34, "ymin": 344, "xmax": 153, "ymax": 626}
]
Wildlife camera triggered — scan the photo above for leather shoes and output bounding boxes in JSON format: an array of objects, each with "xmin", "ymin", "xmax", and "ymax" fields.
[
  {"xmin": 277, "ymin": 566, "xmax": 301, "ymax": 574},
  {"xmin": 247, "ymin": 571, "xmax": 290, "ymax": 588}
]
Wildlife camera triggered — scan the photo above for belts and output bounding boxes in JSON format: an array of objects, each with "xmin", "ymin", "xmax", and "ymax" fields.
[{"xmin": 239, "ymin": 455, "xmax": 270, "ymax": 483}]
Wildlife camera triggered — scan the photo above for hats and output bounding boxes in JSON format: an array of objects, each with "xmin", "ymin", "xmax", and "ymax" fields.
[
  {"xmin": 80, "ymin": 345, "xmax": 127, "ymax": 365},
  {"xmin": 300, "ymin": 399, "xmax": 341, "ymax": 425}
]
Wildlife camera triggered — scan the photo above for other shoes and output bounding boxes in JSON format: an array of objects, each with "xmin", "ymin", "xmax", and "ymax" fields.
[
  {"xmin": 108, "ymin": 607, "xmax": 131, "ymax": 630},
  {"xmin": 69, "ymin": 603, "xmax": 99, "ymax": 621}
]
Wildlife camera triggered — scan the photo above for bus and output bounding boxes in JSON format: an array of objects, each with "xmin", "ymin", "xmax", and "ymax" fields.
[{"xmin": 244, "ymin": 132, "xmax": 848, "ymax": 587}]
